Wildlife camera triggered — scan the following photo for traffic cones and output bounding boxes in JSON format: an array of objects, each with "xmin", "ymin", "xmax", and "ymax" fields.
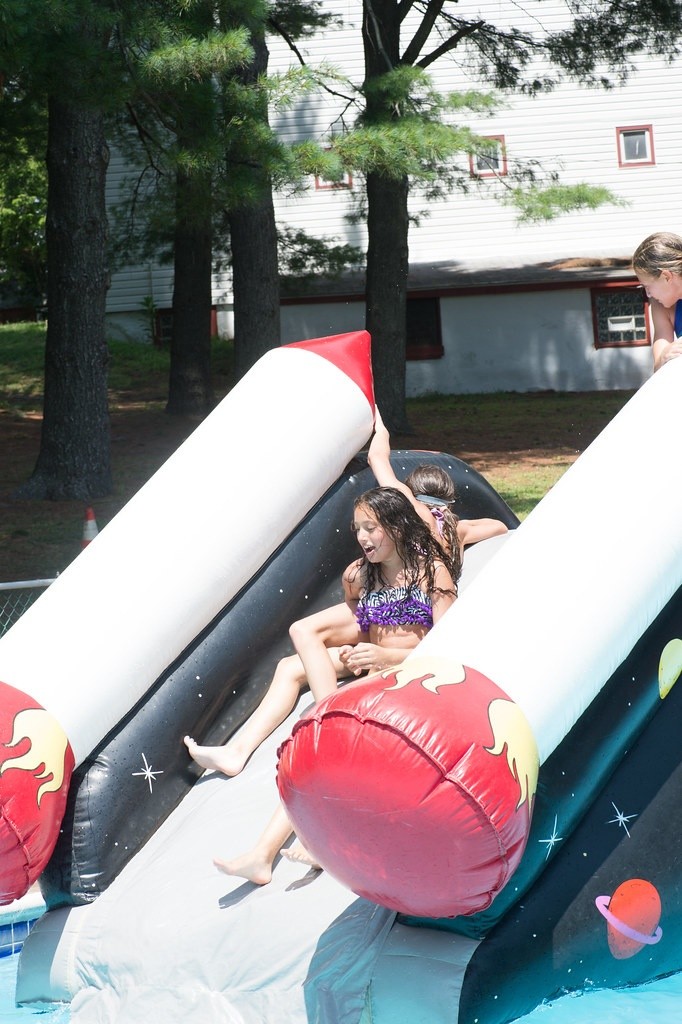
[{"xmin": 79, "ymin": 506, "xmax": 101, "ymax": 551}]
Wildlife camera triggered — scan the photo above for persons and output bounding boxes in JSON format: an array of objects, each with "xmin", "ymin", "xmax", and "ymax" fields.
[
  {"xmin": 184, "ymin": 402, "xmax": 508, "ymax": 776},
  {"xmin": 631, "ymin": 231, "xmax": 682, "ymax": 373},
  {"xmin": 212, "ymin": 486, "xmax": 457, "ymax": 884}
]
